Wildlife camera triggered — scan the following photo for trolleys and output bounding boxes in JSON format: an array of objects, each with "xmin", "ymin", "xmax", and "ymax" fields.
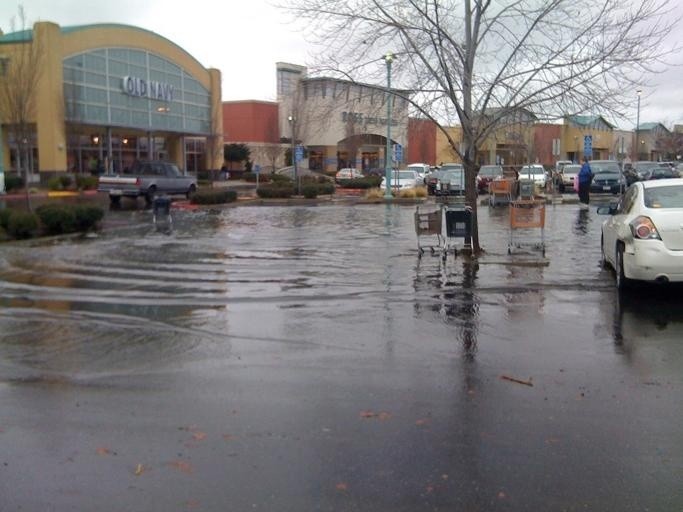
[
  {"xmin": 413, "ymin": 203, "xmax": 447, "ymax": 261},
  {"xmin": 508, "ymin": 196, "xmax": 546, "ymax": 256},
  {"xmin": 442, "ymin": 205, "xmax": 475, "ymax": 263},
  {"xmin": 488, "ymin": 179, "xmax": 512, "ymax": 209}
]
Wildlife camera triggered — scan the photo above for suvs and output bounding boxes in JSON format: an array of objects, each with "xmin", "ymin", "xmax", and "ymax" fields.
[{"xmin": 476, "ymin": 164, "xmax": 521, "ymax": 195}]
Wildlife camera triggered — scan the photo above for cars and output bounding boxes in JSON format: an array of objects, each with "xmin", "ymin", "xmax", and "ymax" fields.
[
  {"xmin": 597, "ymin": 178, "xmax": 683, "ymax": 288},
  {"xmin": 380, "ymin": 163, "xmax": 465, "ymax": 195},
  {"xmin": 550, "ymin": 160, "xmax": 683, "ymax": 195},
  {"xmin": 517, "ymin": 163, "xmax": 550, "ymax": 190},
  {"xmin": 335, "ymin": 168, "xmax": 364, "ymax": 181}
]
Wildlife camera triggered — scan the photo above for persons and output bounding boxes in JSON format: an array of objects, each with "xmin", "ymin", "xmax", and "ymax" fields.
[{"xmin": 572, "ymin": 156, "xmax": 594, "ymax": 207}]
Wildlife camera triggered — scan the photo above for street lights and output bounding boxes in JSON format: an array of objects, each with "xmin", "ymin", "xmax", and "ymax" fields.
[
  {"xmin": 573, "ymin": 135, "xmax": 579, "ymax": 161},
  {"xmin": 635, "ymin": 89, "xmax": 642, "ymax": 155},
  {"xmin": 384, "ymin": 51, "xmax": 394, "ymax": 198},
  {"xmin": 288, "ymin": 115, "xmax": 297, "ymax": 164}
]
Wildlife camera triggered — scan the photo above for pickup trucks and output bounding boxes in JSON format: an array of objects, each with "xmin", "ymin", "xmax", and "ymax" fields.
[{"xmin": 97, "ymin": 161, "xmax": 199, "ymax": 204}]
